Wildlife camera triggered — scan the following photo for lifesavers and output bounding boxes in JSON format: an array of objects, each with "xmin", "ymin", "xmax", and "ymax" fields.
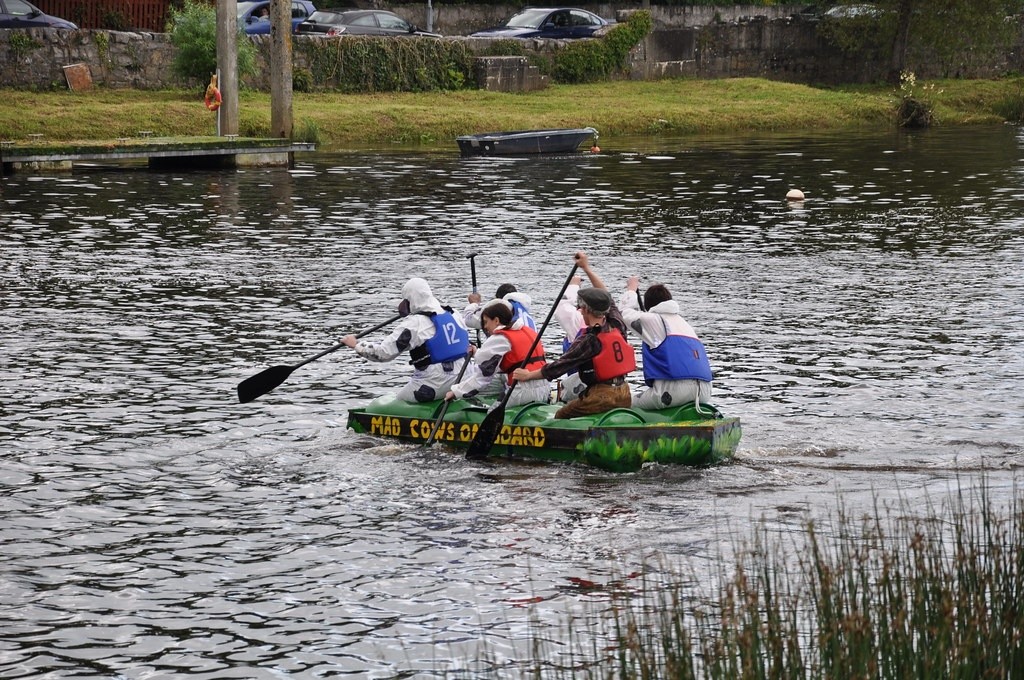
[{"xmin": 205, "ymin": 87, "xmax": 222, "ymax": 110}]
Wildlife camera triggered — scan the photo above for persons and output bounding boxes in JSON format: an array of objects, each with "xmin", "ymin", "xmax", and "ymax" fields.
[
  {"xmin": 342, "ymin": 277, "xmax": 473, "ymax": 405},
  {"xmin": 464, "ymin": 283, "xmax": 537, "ymax": 333},
  {"xmin": 512, "ymin": 253, "xmax": 631, "ymax": 418},
  {"xmin": 618, "ymin": 278, "xmax": 712, "ymax": 411},
  {"xmin": 445, "ymin": 302, "xmax": 551, "ymax": 415},
  {"xmin": 551, "ymin": 277, "xmax": 589, "ymax": 404}
]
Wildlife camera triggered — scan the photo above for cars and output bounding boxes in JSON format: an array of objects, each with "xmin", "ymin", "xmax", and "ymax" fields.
[
  {"xmin": 771, "ymin": 3, "xmax": 898, "ymax": 23},
  {"xmin": 467, "ymin": 7, "xmax": 607, "ymax": 41},
  {"xmin": 0, "ymin": 0, "xmax": 78, "ymax": 29},
  {"xmin": 295, "ymin": 7, "xmax": 443, "ymax": 39}
]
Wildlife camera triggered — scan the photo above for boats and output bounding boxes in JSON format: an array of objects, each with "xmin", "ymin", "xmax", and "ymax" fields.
[
  {"xmin": 346, "ymin": 394, "xmax": 743, "ymax": 471},
  {"xmin": 456, "ymin": 127, "xmax": 598, "ymax": 154}
]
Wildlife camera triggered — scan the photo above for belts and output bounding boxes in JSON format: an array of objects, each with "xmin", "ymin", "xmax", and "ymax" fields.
[{"xmin": 593, "ymin": 375, "xmax": 624, "ymax": 386}]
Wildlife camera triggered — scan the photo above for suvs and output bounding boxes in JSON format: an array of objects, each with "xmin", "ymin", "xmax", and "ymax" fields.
[{"xmin": 202, "ymin": 1, "xmax": 316, "ymax": 35}]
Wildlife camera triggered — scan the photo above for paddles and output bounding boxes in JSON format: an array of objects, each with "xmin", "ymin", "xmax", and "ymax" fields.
[
  {"xmin": 469, "ymin": 255, "xmax": 482, "ymax": 350},
  {"xmin": 465, "ymin": 263, "xmax": 579, "ymax": 459},
  {"xmin": 424, "ymin": 352, "xmax": 473, "ymax": 448},
  {"xmin": 237, "ymin": 316, "xmax": 403, "ymax": 405},
  {"xmin": 636, "ymin": 288, "xmax": 645, "ymax": 311}
]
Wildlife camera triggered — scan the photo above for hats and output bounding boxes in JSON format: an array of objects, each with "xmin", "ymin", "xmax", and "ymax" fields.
[{"xmin": 577, "ymin": 287, "xmax": 611, "ymax": 311}]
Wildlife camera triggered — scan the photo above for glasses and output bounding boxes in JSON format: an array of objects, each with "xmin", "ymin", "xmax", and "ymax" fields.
[{"xmin": 576, "ymin": 304, "xmax": 581, "ymax": 310}]
